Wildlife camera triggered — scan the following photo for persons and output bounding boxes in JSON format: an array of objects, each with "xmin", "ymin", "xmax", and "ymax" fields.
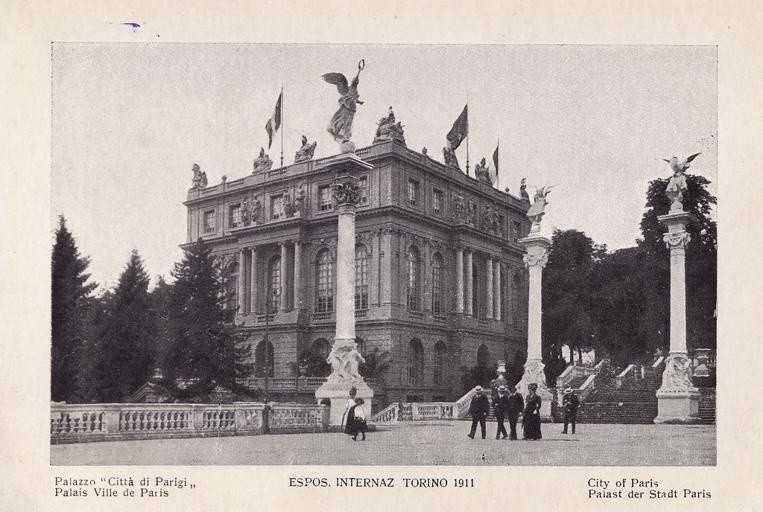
[
  {"xmin": 468, "ymin": 384, "xmax": 489, "ymax": 439},
  {"xmin": 295, "ymin": 135, "xmax": 314, "ymax": 162},
  {"xmin": 252, "ymin": 148, "xmax": 272, "ymax": 174},
  {"xmin": 346, "ymin": 387, "xmax": 368, "ymax": 440},
  {"xmin": 322, "ymin": 72, "xmax": 364, "ymax": 143},
  {"xmin": 561, "ymin": 388, "xmax": 579, "ymax": 434},
  {"xmin": 491, "ymin": 378, "xmax": 541, "ymax": 441},
  {"xmin": 475, "ymin": 158, "xmax": 492, "ymax": 185}
]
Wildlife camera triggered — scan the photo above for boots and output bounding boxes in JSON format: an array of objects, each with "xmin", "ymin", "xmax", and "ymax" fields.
[
  {"xmin": 362, "ymin": 434, "xmax": 365, "ymax": 440},
  {"xmin": 352, "ymin": 435, "xmax": 357, "ymax": 441}
]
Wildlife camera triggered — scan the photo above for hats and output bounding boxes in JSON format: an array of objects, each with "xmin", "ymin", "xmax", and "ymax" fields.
[
  {"xmin": 354, "ymin": 397, "xmax": 364, "ymax": 404},
  {"xmin": 475, "ymin": 385, "xmax": 483, "ymax": 392},
  {"xmin": 563, "ymin": 388, "xmax": 573, "ymax": 393},
  {"xmin": 496, "ymin": 386, "xmax": 506, "ymax": 392}
]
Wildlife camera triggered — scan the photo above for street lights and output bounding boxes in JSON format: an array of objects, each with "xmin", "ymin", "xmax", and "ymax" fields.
[{"xmin": 257, "ymin": 282, "xmax": 282, "ymax": 435}]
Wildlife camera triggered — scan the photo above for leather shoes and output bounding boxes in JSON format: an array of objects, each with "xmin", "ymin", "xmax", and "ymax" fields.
[
  {"xmin": 467, "ymin": 433, "xmax": 538, "ymax": 441},
  {"xmin": 561, "ymin": 431, "xmax": 567, "ymax": 433}
]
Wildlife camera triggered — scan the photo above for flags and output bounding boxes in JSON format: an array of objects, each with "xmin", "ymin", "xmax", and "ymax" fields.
[
  {"xmin": 446, "ymin": 106, "xmax": 468, "ymax": 149},
  {"xmin": 493, "ymin": 147, "xmax": 498, "ymax": 175},
  {"xmin": 264, "ymin": 94, "xmax": 281, "ymax": 149}
]
